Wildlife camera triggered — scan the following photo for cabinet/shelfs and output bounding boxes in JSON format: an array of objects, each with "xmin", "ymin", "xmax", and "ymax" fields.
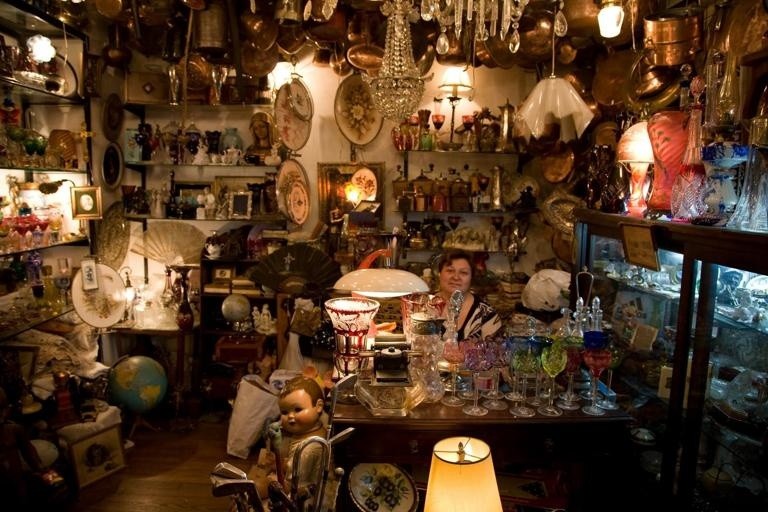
[
  {"xmin": 576, "ymin": 207, "xmax": 767, "ymax": 512},
  {"xmin": 0, "ymin": 0, "xmax": 295, "ymax": 492},
  {"xmin": 392, "ymin": 139, "xmax": 530, "ymax": 259}
]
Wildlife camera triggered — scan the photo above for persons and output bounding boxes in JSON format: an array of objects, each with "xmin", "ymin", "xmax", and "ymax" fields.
[
  {"xmin": 254, "ymin": 373, "xmax": 329, "ymax": 512},
  {"xmin": 246, "ymin": 111, "xmax": 275, "ymax": 164},
  {"xmin": 717, "ymin": 268, "xmax": 742, "ymax": 305},
  {"xmin": 424, "ymin": 245, "xmax": 503, "ymax": 344}
]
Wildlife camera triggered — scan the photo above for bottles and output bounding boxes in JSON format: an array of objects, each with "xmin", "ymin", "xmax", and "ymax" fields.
[
  {"xmin": 671, "ymin": 109, "xmax": 706, "ymax": 217},
  {"xmin": 381, "ymin": 346, "xmax": 402, "ymax": 370},
  {"xmin": 122, "ymin": 268, "xmax": 202, "ymax": 331},
  {"xmin": 48, "ymin": 370, "xmax": 96, "ymax": 429},
  {"xmin": 726, "ymin": 105, "xmax": 767, "ymax": 231},
  {"xmin": 131, "ymin": 121, "xmax": 246, "ymax": 166}
]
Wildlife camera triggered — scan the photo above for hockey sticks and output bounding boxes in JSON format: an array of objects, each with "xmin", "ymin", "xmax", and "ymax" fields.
[{"xmin": 209, "ymin": 373, "xmax": 357, "ymax": 512}]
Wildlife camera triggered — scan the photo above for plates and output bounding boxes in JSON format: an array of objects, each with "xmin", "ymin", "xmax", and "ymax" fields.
[
  {"xmin": 348, "ymin": 461, "xmax": 418, "ymax": 510},
  {"xmin": 101, "ymin": 142, "xmax": 126, "ymax": 191},
  {"xmin": 95, "ymin": 202, "xmax": 132, "ymax": 270},
  {"xmin": 101, "ymin": 93, "xmax": 126, "ymax": 140},
  {"xmin": 273, "ymin": 73, "xmax": 385, "ymax": 224}
]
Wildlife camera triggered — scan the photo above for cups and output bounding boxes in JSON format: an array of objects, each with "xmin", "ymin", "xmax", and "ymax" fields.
[
  {"xmin": 400, "ymin": 294, "xmax": 445, "ymax": 347},
  {"xmin": 323, "ymin": 299, "xmax": 379, "ymax": 374},
  {"xmin": 121, "ymin": 184, "xmax": 136, "ymax": 197}
]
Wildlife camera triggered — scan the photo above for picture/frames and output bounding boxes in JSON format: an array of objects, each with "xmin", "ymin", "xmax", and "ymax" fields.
[{"xmin": 315, "ymin": 160, "xmax": 386, "ymax": 228}]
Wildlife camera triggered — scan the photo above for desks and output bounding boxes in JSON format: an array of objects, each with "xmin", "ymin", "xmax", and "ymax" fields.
[{"xmin": 327, "ymin": 373, "xmax": 637, "ymax": 508}]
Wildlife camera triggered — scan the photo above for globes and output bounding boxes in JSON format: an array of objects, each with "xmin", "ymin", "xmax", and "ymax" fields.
[
  {"xmin": 107, "ymin": 356, "xmax": 168, "ymax": 441},
  {"xmin": 221, "ymin": 293, "xmax": 251, "ymax": 344}
]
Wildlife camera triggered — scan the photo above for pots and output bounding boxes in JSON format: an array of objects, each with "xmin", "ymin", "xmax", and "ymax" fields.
[{"xmin": 40, "ymin": 0, "xmax": 765, "ymax": 114}]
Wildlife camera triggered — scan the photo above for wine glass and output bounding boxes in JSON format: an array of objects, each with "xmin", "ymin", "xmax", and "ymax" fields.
[
  {"xmin": 1, "ymin": 196, "xmax": 79, "ymax": 253},
  {"xmin": 394, "ymin": 98, "xmax": 519, "ymax": 252},
  {"xmin": 0, "ymin": 122, "xmax": 79, "ymax": 169},
  {"xmin": 7, "ymin": 257, "xmax": 74, "ymax": 323},
  {"xmin": 441, "ymin": 332, "xmax": 623, "ymax": 418}
]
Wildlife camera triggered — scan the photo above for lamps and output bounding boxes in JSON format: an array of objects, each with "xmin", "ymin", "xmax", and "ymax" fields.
[
  {"xmin": 247, "ymin": 0, "xmax": 574, "ymax": 138},
  {"xmin": 334, "ymin": 247, "xmax": 429, "ymax": 345},
  {"xmin": 424, "ymin": 0, "xmax": 658, "ymax": 217},
  {"xmin": 322, "ymin": 295, "xmax": 380, "ymax": 406},
  {"xmin": 422, "ymin": 436, "xmax": 507, "ymax": 512}
]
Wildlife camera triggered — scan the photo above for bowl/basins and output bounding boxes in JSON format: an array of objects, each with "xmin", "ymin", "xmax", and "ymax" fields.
[{"xmin": 14, "ymin": 71, "xmax": 64, "ymax": 92}]
